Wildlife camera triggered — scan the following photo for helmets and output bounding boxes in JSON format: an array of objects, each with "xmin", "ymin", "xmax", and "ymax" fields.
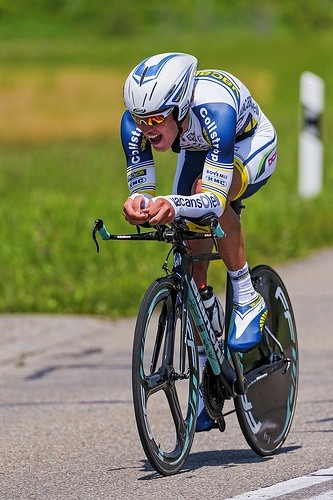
[{"xmin": 123, "ymin": 52, "xmax": 198, "ymax": 121}]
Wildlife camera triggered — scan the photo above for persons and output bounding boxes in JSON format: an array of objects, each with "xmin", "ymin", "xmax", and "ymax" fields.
[{"xmin": 119, "ymin": 51, "xmax": 278, "ymax": 434}]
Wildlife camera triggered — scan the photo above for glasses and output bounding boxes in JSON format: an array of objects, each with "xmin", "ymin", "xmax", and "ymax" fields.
[{"xmin": 129, "ymin": 107, "xmax": 176, "ymax": 125}]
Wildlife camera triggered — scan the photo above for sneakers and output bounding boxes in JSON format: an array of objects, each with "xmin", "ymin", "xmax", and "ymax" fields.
[
  {"xmin": 229, "ymin": 292, "xmax": 269, "ymax": 353},
  {"xmin": 196, "ymin": 382, "xmax": 213, "ymax": 432}
]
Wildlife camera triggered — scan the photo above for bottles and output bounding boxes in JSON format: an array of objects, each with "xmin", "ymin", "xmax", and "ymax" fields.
[{"xmin": 198, "ymin": 283, "xmax": 225, "ymax": 336}]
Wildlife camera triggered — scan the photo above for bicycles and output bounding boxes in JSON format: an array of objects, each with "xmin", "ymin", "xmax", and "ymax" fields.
[{"xmin": 91, "ymin": 212, "xmax": 301, "ymax": 476}]
